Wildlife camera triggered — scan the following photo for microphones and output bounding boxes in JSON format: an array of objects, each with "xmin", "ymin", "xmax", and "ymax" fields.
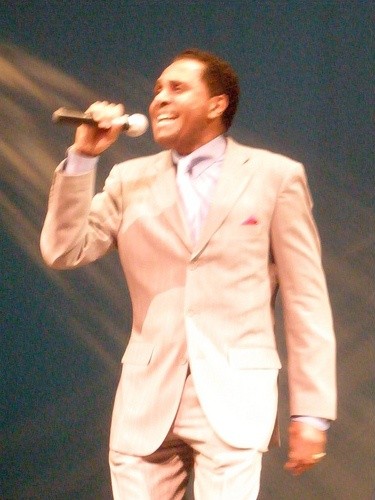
[{"xmin": 52, "ymin": 108, "xmax": 149, "ymax": 138}]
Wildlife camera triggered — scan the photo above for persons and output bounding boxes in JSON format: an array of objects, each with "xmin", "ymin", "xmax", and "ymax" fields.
[{"xmin": 40, "ymin": 49, "xmax": 338, "ymax": 500}]
